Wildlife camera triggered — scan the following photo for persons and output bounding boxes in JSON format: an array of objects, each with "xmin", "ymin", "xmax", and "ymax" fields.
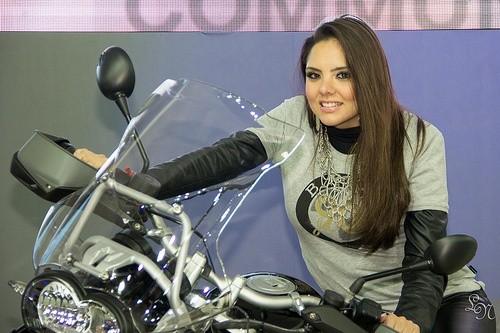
[{"xmin": 67, "ymin": 13, "xmax": 498, "ymax": 333}]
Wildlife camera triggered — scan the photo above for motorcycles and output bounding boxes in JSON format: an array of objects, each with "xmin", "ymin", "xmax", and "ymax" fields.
[{"xmin": 6, "ymin": 45, "xmax": 479, "ymax": 333}]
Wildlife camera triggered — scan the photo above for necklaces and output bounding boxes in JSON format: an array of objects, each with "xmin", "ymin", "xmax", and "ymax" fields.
[{"xmin": 314, "ymin": 121, "xmax": 366, "ymax": 224}]
